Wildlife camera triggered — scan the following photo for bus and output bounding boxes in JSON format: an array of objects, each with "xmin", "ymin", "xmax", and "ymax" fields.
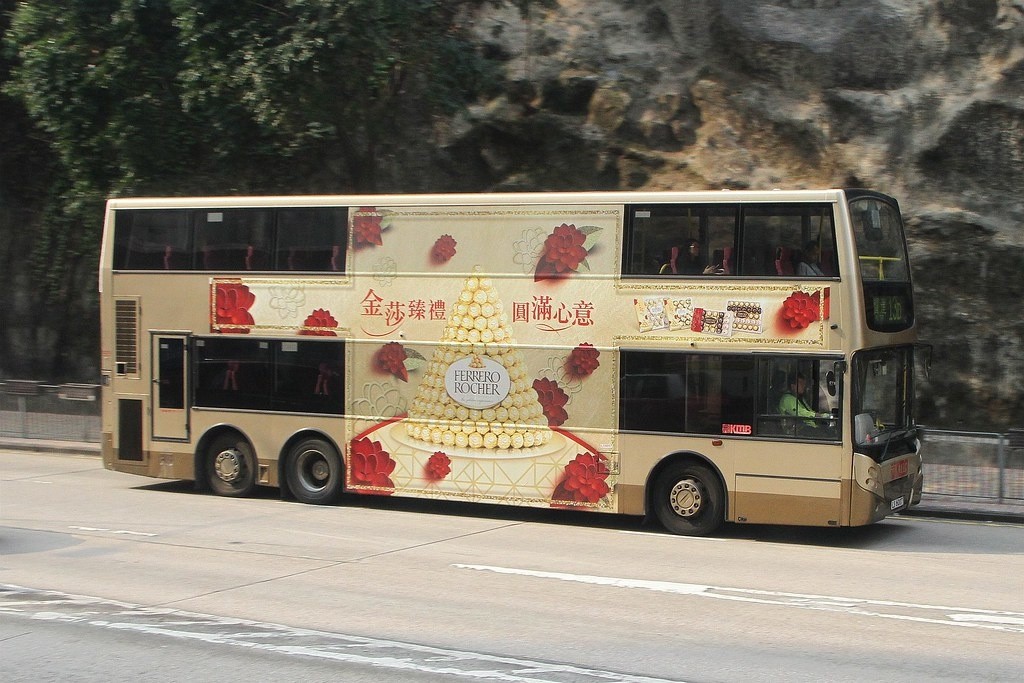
[{"xmin": 98, "ymin": 187, "xmax": 935, "ymax": 538}]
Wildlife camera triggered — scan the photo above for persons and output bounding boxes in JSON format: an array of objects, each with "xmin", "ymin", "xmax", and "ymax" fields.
[
  {"xmin": 676, "ymin": 240, "xmax": 718, "ymax": 274},
  {"xmin": 779, "ymin": 373, "xmax": 828, "ymax": 435},
  {"xmin": 796, "ymin": 242, "xmax": 827, "ymax": 276},
  {"xmin": 650, "ymin": 238, "xmax": 685, "ymax": 275}
]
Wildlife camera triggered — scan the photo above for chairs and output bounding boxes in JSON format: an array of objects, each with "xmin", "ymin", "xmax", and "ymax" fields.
[
  {"xmin": 671, "ymin": 240, "xmax": 834, "ymax": 277},
  {"xmin": 194, "ymin": 361, "xmax": 344, "ymax": 414},
  {"xmin": 163, "ymin": 245, "xmax": 343, "ymax": 271}
]
